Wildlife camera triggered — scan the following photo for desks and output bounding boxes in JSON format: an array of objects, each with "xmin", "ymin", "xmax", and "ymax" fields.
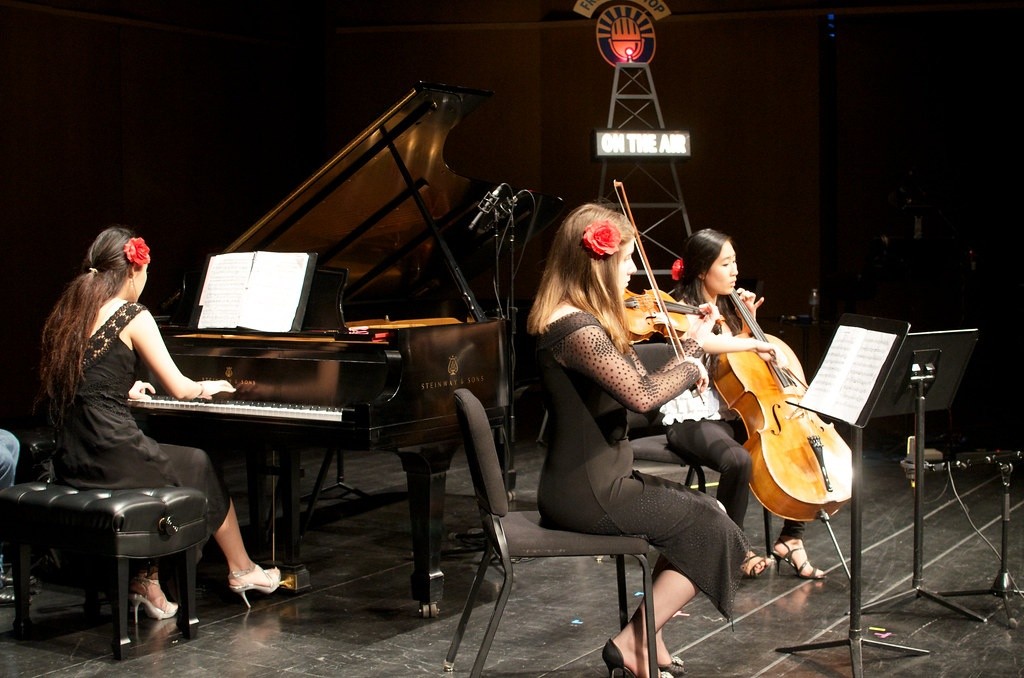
[{"xmin": 777, "ymin": 315, "xmax": 835, "ymax": 382}]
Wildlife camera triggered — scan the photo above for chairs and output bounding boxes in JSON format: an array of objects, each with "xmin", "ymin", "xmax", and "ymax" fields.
[
  {"xmin": 443, "ymin": 389, "xmax": 659, "ymax": 678},
  {"xmin": 626, "ymin": 343, "xmax": 773, "ymax": 559}
]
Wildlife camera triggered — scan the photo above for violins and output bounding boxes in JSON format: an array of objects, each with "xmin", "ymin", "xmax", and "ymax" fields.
[{"xmin": 617, "ymin": 287, "xmax": 712, "ymax": 344}]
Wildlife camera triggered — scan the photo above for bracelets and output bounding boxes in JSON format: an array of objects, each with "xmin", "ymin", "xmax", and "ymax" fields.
[
  {"xmin": 195, "ymin": 381, "xmax": 205, "ymax": 399},
  {"xmin": 39, "ymin": 227, "xmax": 282, "ymax": 624}
]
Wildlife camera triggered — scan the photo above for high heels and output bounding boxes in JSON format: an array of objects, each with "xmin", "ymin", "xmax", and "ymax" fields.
[
  {"xmin": 229, "ymin": 565, "xmax": 281, "ymax": 608},
  {"xmin": 742, "ymin": 555, "xmax": 771, "ymax": 578},
  {"xmin": 602, "ymin": 638, "xmax": 688, "ymax": 678},
  {"xmin": 771, "ymin": 537, "xmax": 826, "ymax": 579},
  {"xmin": 129, "ymin": 576, "xmax": 178, "ymax": 624}
]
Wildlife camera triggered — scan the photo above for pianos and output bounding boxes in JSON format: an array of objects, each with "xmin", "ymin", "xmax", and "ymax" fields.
[{"xmin": 121, "ymin": 79, "xmax": 519, "ymax": 632}]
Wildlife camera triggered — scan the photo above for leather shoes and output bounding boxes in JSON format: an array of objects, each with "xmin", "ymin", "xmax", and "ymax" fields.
[{"xmin": 0, "ymin": 574, "xmax": 36, "ymax": 607}]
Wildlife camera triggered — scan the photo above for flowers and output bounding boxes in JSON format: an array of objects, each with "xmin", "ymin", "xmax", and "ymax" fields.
[
  {"xmin": 582, "ymin": 220, "xmax": 621, "ymax": 256},
  {"xmin": 672, "ymin": 259, "xmax": 684, "ymax": 281}
]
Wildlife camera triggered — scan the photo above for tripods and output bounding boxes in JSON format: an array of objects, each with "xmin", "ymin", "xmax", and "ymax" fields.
[{"xmin": 776, "ymin": 312, "xmax": 1024, "ymax": 678}]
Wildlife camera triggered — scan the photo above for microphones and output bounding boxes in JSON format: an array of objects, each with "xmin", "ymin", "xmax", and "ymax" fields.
[
  {"xmin": 468, "ymin": 186, "xmax": 502, "ymax": 229},
  {"xmin": 906, "ymin": 435, "xmax": 916, "ymax": 488},
  {"xmin": 483, "ymin": 194, "xmax": 521, "ymax": 232}
]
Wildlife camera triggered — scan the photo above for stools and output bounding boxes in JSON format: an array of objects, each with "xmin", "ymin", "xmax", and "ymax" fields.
[
  {"xmin": 0, "ymin": 480, "xmax": 208, "ymax": 660},
  {"xmin": 11, "ymin": 430, "xmax": 54, "ymax": 480}
]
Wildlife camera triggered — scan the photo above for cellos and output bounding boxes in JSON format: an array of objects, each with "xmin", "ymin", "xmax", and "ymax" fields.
[{"xmin": 707, "ymin": 286, "xmax": 858, "ymax": 589}]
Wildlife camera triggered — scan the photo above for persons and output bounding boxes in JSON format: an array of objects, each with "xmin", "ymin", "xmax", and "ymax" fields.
[
  {"xmin": 0, "ymin": 429, "xmax": 38, "ymax": 607},
  {"xmin": 660, "ymin": 228, "xmax": 828, "ymax": 580},
  {"xmin": 526, "ymin": 202, "xmax": 752, "ymax": 678}
]
